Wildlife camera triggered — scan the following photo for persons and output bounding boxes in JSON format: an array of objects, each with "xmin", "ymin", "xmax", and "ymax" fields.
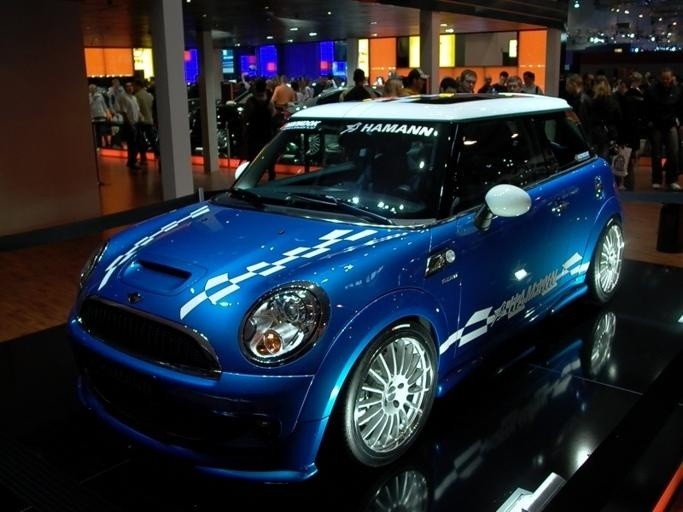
[
  {"xmin": 265, "ymin": 74, "xmax": 334, "ymax": 119},
  {"xmin": 345, "ymin": 69, "xmax": 370, "ymax": 101},
  {"xmin": 244, "ymin": 79, "xmax": 275, "ymax": 180},
  {"xmin": 439, "ymin": 69, "xmax": 477, "ymax": 93},
  {"xmin": 559, "ymin": 67, "xmax": 682, "ymax": 192},
  {"xmin": 478, "ymin": 71, "xmax": 543, "ymax": 95},
  {"xmin": 89, "ymin": 77, "xmax": 159, "ymax": 167},
  {"xmin": 383, "ymin": 69, "xmax": 427, "ymax": 98}
]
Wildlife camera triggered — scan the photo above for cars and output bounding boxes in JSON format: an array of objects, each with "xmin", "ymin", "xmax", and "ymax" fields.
[
  {"xmin": 58, "ymin": 89, "xmax": 626, "ymax": 485},
  {"xmin": 147, "ymin": 86, "xmax": 348, "ymax": 162},
  {"xmin": 351, "ymin": 302, "xmax": 619, "ymax": 508}
]
